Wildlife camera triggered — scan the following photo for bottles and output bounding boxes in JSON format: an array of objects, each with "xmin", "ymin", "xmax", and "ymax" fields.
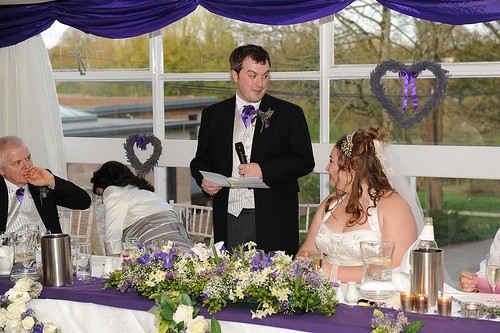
[
  {"xmin": 345, "ymin": 282, "xmax": 360, "ymax": 302},
  {"xmin": 102, "ymin": 258, "xmax": 113, "ymax": 275},
  {"xmin": 417, "ymin": 217, "xmax": 438, "ymax": 254}
]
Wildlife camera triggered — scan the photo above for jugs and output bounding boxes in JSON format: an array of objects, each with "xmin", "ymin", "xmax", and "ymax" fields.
[
  {"xmin": 358, "ymin": 241, "xmax": 396, "ymax": 300},
  {"xmin": 9, "ymin": 223, "xmax": 42, "ymax": 284}
]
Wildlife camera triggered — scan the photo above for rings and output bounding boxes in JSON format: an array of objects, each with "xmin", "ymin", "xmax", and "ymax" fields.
[{"xmin": 210, "ymin": 192, "xmax": 213, "ymax": 195}]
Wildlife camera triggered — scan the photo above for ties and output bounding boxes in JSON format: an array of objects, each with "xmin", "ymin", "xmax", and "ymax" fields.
[
  {"xmin": 16, "ymin": 188, "xmax": 25, "ymax": 204},
  {"xmin": 241, "ymin": 104, "xmax": 255, "ymax": 128}
]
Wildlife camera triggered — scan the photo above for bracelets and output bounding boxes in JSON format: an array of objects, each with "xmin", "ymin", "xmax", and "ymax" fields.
[{"xmin": 329, "ymin": 264, "xmax": 338, "ymax": 285}]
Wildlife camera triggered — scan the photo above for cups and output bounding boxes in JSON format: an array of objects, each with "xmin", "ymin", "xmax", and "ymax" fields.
[
  {"xmin": 253, "ymin": 250, "xmax": 278, "ymax": 261},
  {"xmin": 123, "ymin": 236, "xmax": 165, "ymax": 257},
  {"xmin": 0, "ymin": 230, "xmax": 92, "ymax": 282}
]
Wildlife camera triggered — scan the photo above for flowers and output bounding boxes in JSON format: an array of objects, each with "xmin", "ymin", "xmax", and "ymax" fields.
[
  {"xmin": 107, "ymin": 239, "xmax": 425, "ymax": 333},
  {"xmin": 241, "ymin": 105, "xmax": 274, "ymax": 133},
  {"xmin": 0, "ymin": 279, "xmax": 62, "ymax": 333}
]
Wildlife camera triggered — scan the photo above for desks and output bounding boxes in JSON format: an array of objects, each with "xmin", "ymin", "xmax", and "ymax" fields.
[{"xmin": 0, "ymin": 265, "xmax": 500, "ymax": 333}]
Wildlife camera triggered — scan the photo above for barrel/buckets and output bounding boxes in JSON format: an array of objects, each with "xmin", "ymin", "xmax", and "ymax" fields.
[
  {"xmin": 41, "ymin": 234, "xmax": 72, "ymax": 287},
  {"xmin": 411, "ymin": 249, "xmax": 445, "ymax": 306}
]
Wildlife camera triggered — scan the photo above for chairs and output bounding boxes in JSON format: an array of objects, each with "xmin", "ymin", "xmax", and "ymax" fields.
[
  {"xmin": 57, "ymin": 204, "xmax": 94, "ymax": 255},
  {"xmin": 168, "ymin": 204, "xmax": 215, "ymax": 247}
]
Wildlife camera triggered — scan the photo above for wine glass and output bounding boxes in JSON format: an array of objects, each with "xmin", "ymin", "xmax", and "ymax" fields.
[{"xmin": 485, "ymin": 254, "xmax": 500, "ymax": 304}]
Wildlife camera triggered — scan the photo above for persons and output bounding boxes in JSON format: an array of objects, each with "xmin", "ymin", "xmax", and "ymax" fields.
[
  {"xmin": 292, "ymin": 127, "xmax": 418, "ymax": 292},
  {"xmin": 190, "ymin": 44, "xmax": 316, "ymax": 263},
  {"xmin": 0, "ymin": 135, "xmax": 91, "ymax": 246},
  {"xmin": 89, "ymin": 160, "xmax": 192, "ymax": 257},
  {"xmin": 458, "ymin": 225, "xmax": 500, "ymax": 295}
]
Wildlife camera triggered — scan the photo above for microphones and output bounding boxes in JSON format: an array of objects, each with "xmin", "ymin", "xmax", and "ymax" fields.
[{"xmin": 235, "ymin": 141, "xmax": 248, "ymax": 176}]
